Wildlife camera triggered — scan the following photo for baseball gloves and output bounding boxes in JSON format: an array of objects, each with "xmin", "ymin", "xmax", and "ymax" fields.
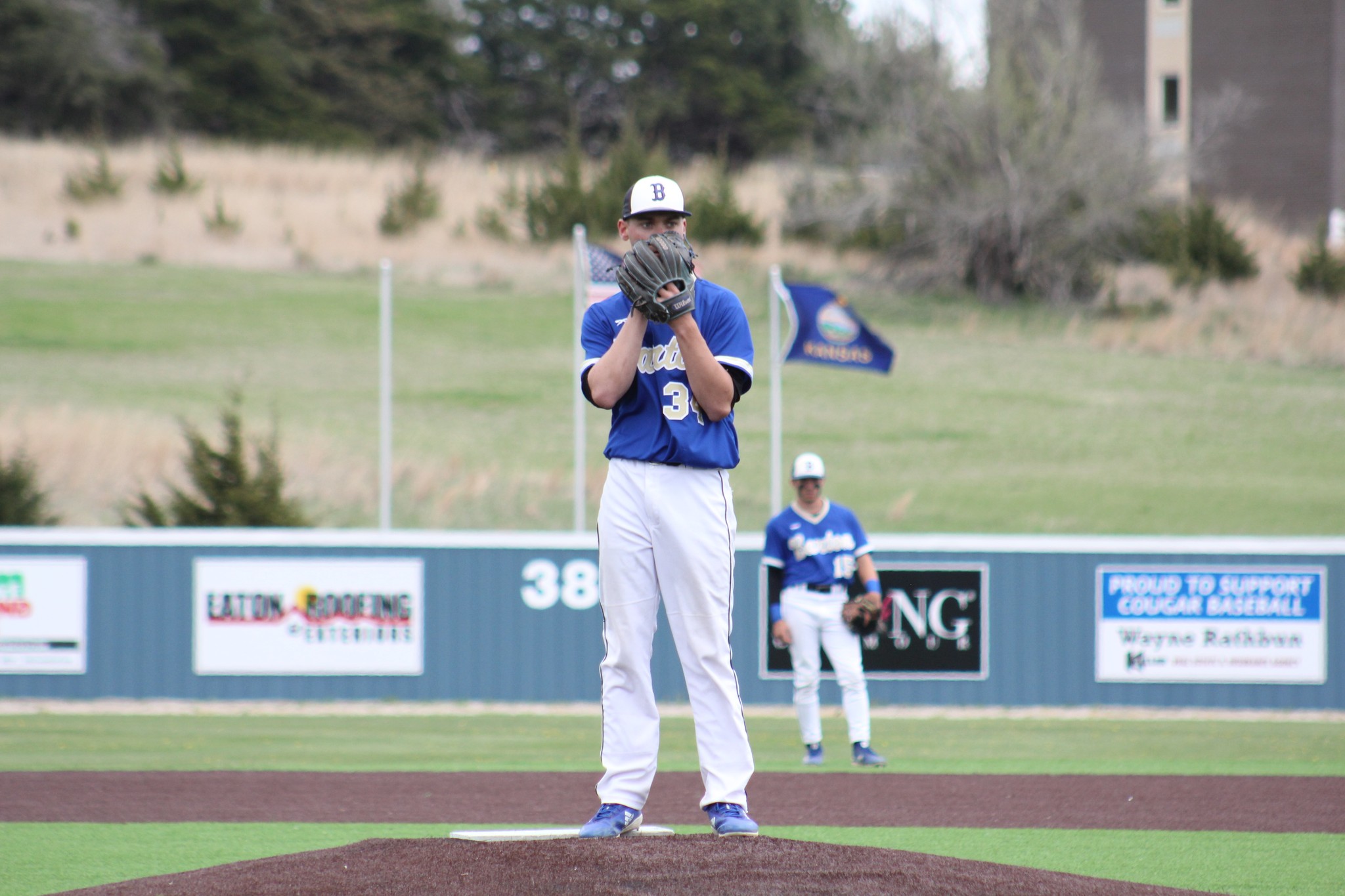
[
  {"xmin": 842, "ymin": 595, "xmax": 882, "ymax": 636},
  {"xmin": 615, "ymin": 231, "xmax": 695, "ymax": 325}
]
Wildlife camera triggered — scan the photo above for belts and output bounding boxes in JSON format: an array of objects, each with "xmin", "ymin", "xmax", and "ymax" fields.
[
  {"xmin": 649, "ymin": 461, "xmax": 681, "ymax": 466},
  {"xmin": 807, "ymin": 582, "xmax": 830, "ymax": 594}
]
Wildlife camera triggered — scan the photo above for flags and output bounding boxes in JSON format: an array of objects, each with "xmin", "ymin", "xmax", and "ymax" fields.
[
  {"xmin": 582, "ymin": 243, "xmax": 624, "ymax": 293},
  {"xmin": 771, "ymin": 266, "xmax": 893, "ymax": 376}
]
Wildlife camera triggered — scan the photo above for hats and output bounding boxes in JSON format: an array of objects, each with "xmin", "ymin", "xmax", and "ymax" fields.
[
  {"xmin": 623, "ymin": 176, "xmax": 691, "ymax": 220},
  {"xmin": 792, "ymin": 453, "xmax": 825, "ymax": 480}
]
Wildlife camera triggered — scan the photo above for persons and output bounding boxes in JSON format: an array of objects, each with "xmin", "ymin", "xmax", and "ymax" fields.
[
  {"xmin": 759, "ymin": 453, "xmax": 888, "ymax": 767},
  {"xmin": 580, "ymin": 175, "xmax": 755, "ymax": 839}
]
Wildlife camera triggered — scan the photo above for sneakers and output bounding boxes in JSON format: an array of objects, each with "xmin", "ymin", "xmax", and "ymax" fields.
[
  {"xmin": 707, "ymin": 803, "xmax": 758, "ymax": 837},
  {"xmin": 804, "ymin": 744, "xmax": 823, "ymax": 765},
  {"xmin": 853, "ymin": 742, "xmax": 885, "ymax": 765},
  {"xmin": 578, "ymin": 804, "xmax": 643, "ymax": 840}
]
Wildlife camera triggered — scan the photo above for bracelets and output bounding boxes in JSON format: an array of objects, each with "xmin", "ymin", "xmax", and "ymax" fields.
[{"xmin": 865, "ymin": 580, "xmax": 882, "ymax": 596}]
